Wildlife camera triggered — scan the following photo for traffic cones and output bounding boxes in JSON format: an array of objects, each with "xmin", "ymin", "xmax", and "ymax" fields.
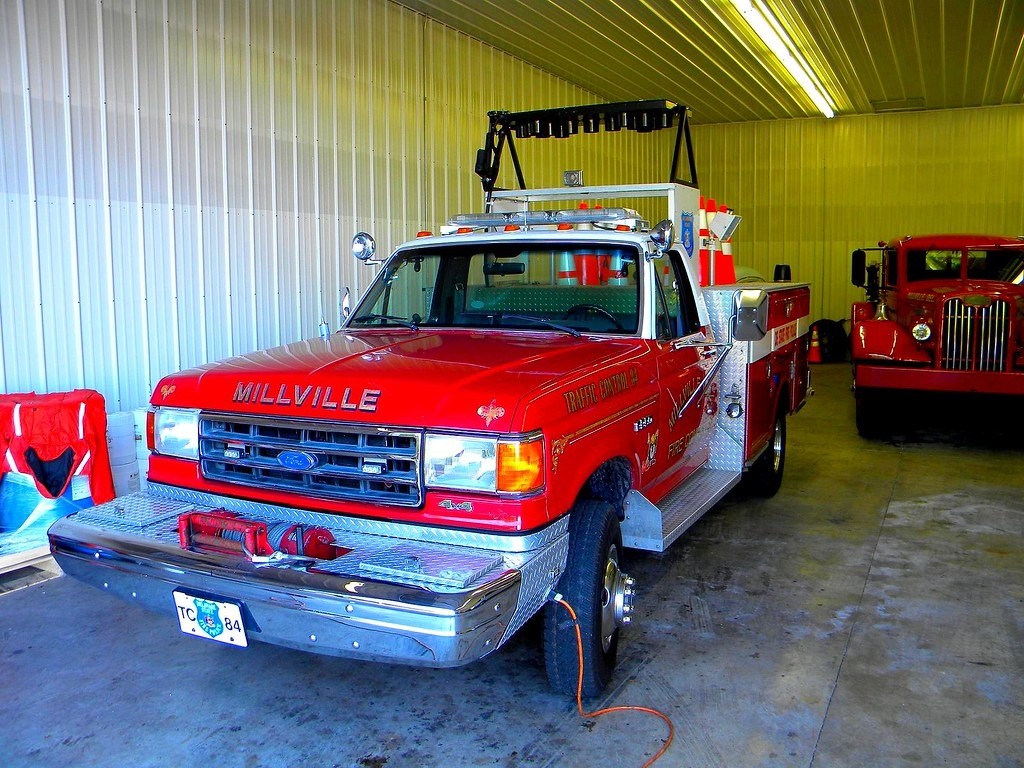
[
  {"xmin": 555, "ymin": 250, "xmax": 579, "ymax": 285},
  {"xmin": 606, "ymin": 249, "xmax": 630, "ymax": 286},
  {"xmin": 592, "ymin": 205, "xmax": 611, "ymax": 281},
  {"xmin": 806, "ymin": 325, "xmax": 823, "ymax": 363},
  {"xmin": 574, "ymin": 202, "xmax": 601, "ymax": 286},
  {"xmin": 699, "ymin": 195, "xmax": 715, "ymax": 288},
  {"xmin": 705, "ymin": 197, "xmax": 738, "ymax": 284}
]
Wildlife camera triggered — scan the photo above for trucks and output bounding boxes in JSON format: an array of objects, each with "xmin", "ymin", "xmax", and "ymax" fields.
[{"xmin": 846, "ymin": 233, "xmax": 1024, "ymax": 448}]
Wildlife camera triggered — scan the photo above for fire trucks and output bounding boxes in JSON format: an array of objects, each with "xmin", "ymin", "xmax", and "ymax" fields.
[{"xmin": 46, "ymin": 95, "xmax": 818, "ymax": 699}]
